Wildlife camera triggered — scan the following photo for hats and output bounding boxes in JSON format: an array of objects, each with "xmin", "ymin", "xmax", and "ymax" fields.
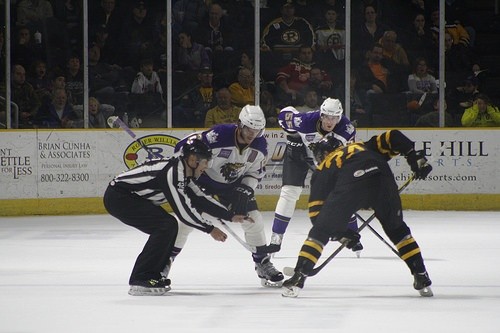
[
  {"xmin": 280, "ymin": 0, "xmax": 296, "ymax": 8},
  {"xmin": 129, "ymin": 0, "xmax": 148, "ymax": 9},
  {"xmin": 89, "ymin": 38, "xmax": 103, "ymax": 49}
]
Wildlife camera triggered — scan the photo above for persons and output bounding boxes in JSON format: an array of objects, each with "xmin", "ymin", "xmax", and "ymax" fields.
[
  {"xmin": 282, "ymin": 129, "xmax": 433, "ymax": 297},
  {"xmin": 103, "ymin": 139, "xmax": 255, "ymax": 296},
  {"xmin": 0, "ymin": 0, "xmax": 500, "ymax": 129},
  {"xmin": 161, "ymin": 104, "xmax": 284, "ymax": 288},
  {"xmin": 267, "ymin": 97, "xmax": 363, "ymax": 259}
]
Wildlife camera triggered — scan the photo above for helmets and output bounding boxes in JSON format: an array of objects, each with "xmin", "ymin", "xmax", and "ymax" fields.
[
  {"xmin": 320, "ymin": 97, "xmax": 343, "ymax": 124},
  {"xmin": 181, "ymin": 139, "xmax": 213, "ymax": 168},
  {"xmin": 312, "ymin": 136, "xmax": 344, "ymax": 167},
  {"xmin": 238, "ymin": 105, "xmax": 266, "ymax": 138},
  {"xmin": 198, "ymin": 64, "xmax": 213, "ymax": 71}
]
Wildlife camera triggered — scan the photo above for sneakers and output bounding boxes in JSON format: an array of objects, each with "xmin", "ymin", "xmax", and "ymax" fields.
[
  {"xmin": 414, "ymin": 266, "xmax": 433, "ymax": 297},
  {"xmin": 265, "ymin": 237, "xmax": 281, "ymax": 254},
  {"xmin": 351, "ymin": 241, "xmax": 363, "ymax": 258},
  {"xmin": 281, "ymin": 271, "xmax": 307, "ymax": 297},
  {"xmin": 128, "ymin": 273, "xmax": 171, "ymax": 295},
  {"xmin": 255, "ymin": 256, "xmax": 284, "ymax": 288},
  {"xmin": 160, "ymin": 257, "xmax": 174, "ymax": 285}
]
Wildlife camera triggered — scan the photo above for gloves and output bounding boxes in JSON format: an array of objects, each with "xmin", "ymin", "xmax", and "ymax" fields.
[
  {"xmin": 286, "ymin": 130, "xmax": 308, "ymax": 161},
  {"xmin": 228, "ymin": 184, "xmax": 253, "ymax": 215},
  {"xmin": 406, "ymin": 148, "xmax": 432, "ymax": 180},
  {"xmin": 186, "ymin": 182, "xmax": 211, "ymax": 209},
  {"xmin": 331, "ymin": 229, "xmax": 361, "ymax": 249}
]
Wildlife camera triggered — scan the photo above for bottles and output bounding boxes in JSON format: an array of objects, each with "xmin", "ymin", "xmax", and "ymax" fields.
[{"xmin": 123, "ymin": 113, "xmax": 128, "ymax": 126}]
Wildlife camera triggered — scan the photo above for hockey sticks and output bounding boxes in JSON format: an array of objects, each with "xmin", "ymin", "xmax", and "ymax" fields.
[
  {"xmin": 300, "ymin": 154, "xmax": 402, "ymax": 260},
  {"xmin": 282, "ymin": 163, "xmax": 428, "ymax": 277},
  {"xmin": 113, "ymin": 115, "xmax": 281, "ymax": 255}
]
